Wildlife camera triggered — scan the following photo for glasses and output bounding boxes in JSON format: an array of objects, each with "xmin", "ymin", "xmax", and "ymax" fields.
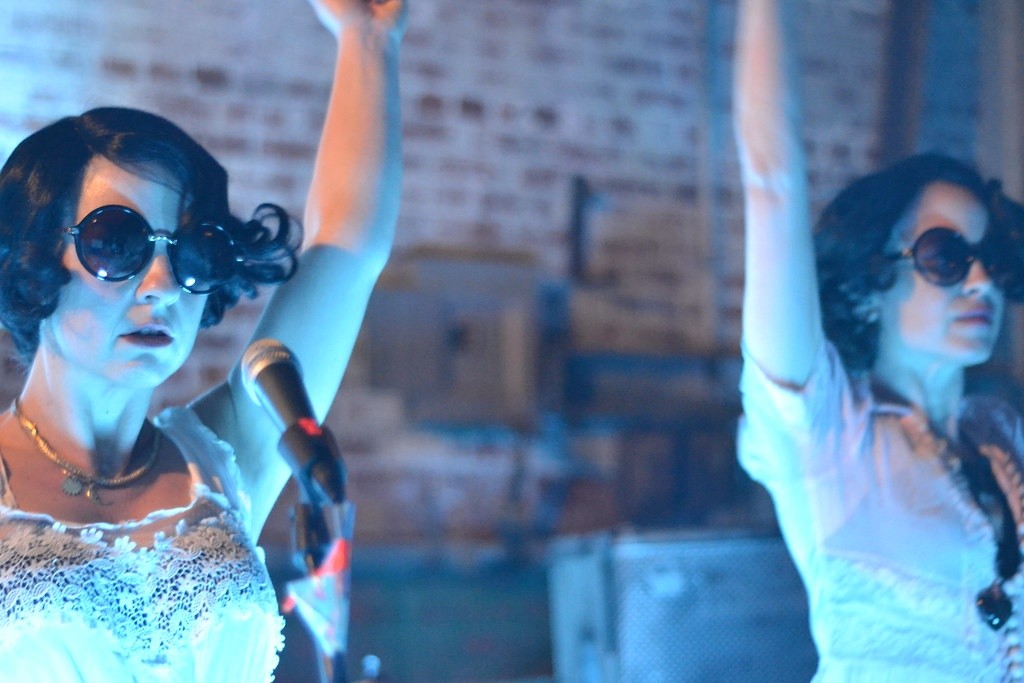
[
  {"xmin": 64, "ymin": 204, "xmax": 245, "ymax": 294},
  {"xmin": 884, "ymin": 227, "xmax": 1017, "ymax": 288}
]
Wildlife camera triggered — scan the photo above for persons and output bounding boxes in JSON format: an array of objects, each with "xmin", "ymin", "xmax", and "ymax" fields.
[
  {"xmin": 735, "ymin": 0, "xmax": 1024, "ymax": 683},
  {"xmin": 0, "ymin": 0, "xmax": 412, "ymax": 683}
]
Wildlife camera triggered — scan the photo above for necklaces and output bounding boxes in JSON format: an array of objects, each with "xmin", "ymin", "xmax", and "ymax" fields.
[
  {"xmin": 10, "ymin": 397, "xmax": 164, "ymax": 506},
  {"xmin": 869, "ymin": 369, "xmax": 1024, "ymax": 632}
]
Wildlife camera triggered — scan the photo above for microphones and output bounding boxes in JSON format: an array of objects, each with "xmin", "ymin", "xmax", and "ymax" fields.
[{"xmin": 241, "ymin": 337, "xmax": 348, "ymax": 503}]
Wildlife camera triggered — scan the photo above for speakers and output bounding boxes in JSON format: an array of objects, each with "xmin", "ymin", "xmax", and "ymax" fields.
[{"xmin": 548, "ymin": 524, "xmax": 820, "ymax": 683}]
